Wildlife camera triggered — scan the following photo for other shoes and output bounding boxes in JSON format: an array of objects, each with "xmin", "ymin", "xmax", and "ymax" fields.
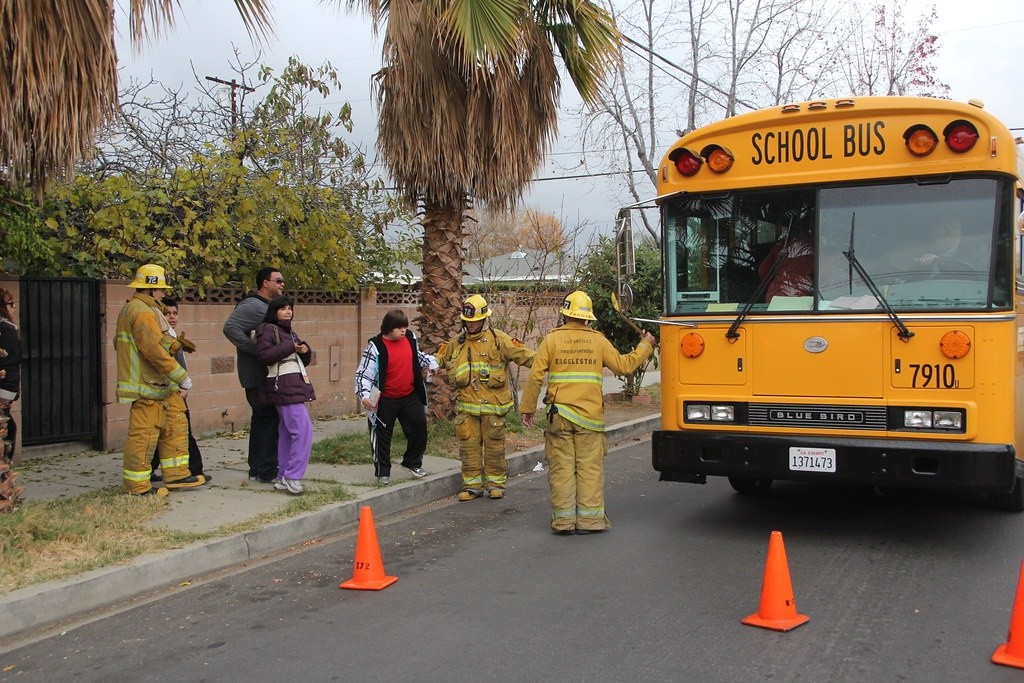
[
  {"xmin": 191, "ymin": 472, "xmax": 212, "ymax": 481},
  {"xmin": 150, "ymin": 474, "xmax": 163, "ymax": 481}
]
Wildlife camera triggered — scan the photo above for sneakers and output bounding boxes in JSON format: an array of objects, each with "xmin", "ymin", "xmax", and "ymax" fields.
[
  {"xmin": 489, "ymin": 490, "xmax": 503, "ymax": 498},
  {"xmin": 378, "ymin": 476, "xmax": 389, "ymax": 484},
  {"xmin": 273, "ymin": 476, "xmax": 303, "ymax": 494},
  {"xmin": 165, "ymin": 475, "xmax": 206, "ymax": 489},
  {"xmin": 399, "ymin": 464, "xmax": 427, "ymax": 478},
  {"xmin": 141, "ymin": 487, "xmax": 169, "ymax": 497},
  {"xmin": 559, "ymin": 529, "xmax": 593, "ymax": 535},
  {"xmin": 458, "ymin": 489, "xmax": 477, "ymax": 500}
]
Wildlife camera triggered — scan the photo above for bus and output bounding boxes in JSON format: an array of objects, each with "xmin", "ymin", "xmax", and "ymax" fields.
[{"xmin": 611, "ymin": 95, "xmax": 1024, "ymax": 512}]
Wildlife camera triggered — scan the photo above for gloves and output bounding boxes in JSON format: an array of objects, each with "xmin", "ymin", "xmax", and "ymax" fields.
[{"xmin": 180, "ymin": 376, "xmax": 192, "ymax": 390}]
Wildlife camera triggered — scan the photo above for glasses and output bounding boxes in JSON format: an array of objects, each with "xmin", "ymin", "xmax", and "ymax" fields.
[
  {"xmin": 270, "ymin": 278, "xmax": 284, "ymax": 284},
  {"xmin": 6, "ymin": 301, "xmax": 14, "ymax": 307}
]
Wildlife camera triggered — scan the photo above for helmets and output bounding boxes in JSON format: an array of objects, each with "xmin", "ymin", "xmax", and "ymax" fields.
[
  {"xmin": 559, "ymin": 291, "xmax": 597, "ymax": 320},
  {"xmin": 126, "ymin": 264, "xmax": 173, "ymax": 289},
  {"xmin": 459, "ymin": 294, "xmax": 492, "ymax": 321}
]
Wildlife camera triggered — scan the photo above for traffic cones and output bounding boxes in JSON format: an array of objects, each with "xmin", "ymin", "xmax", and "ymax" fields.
[
  {"xmin": 339, "ymin": 505, "xmax": 398, "ymax": 591},
  {"xmin": 992, "ymin": 558, "xmax": 1024, "ymax": 668},
  {"xmin": 740, "ymin": 530, "xmax": 810, "ymax": 632}
]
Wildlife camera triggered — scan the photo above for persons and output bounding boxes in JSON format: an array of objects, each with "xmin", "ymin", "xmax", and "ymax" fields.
[
  {"xmin": 429, "ymin": 295, "xmax": 538, "ymax": 501},
  {"xmin": 519, "ymin": 291, "xmax": 656, "ymax": 535},
  {"xmin": 914, "ymin": 216, "xmax": 986, "ymax": 298},
  {"xmin": 113, "ymin": 264, "xmax": 212, "ymax": 496},
  {"xmin": 356, "ymin": 309, "xmax": 440, "ymax": 486},
  {"xmin": 256, "ymin": 295, "xmax": 317, "ymax": 496},
  {"xmin": 758, "ymin": 220, "xmax": 841, "ymax": 304},
  {"xmin": 0, "ymin": 287, "xmax": 21, "ymax": 465},
  {"xmin": 223, "ymin": 267, "xmax": 285, "ymax": 483}
]
